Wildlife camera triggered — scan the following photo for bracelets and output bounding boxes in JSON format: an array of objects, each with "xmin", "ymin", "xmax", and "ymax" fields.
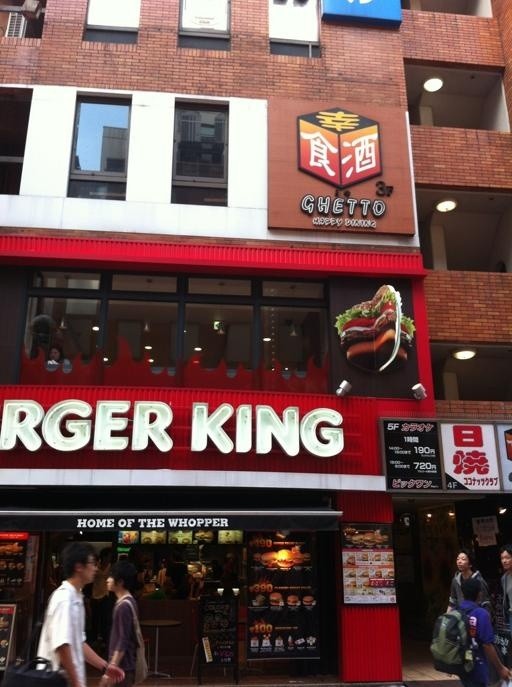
[{"xmin": 102, "ymin": 662, "xmax": 108, "ymax": 673}]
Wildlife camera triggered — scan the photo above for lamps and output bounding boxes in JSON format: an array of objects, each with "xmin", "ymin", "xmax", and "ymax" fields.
[
  {"xmin": 412, "ymin": 384, "xmax": 429, "ymax": 400},
  {"xmin": 335, "ymin": 378, "xmax": 352, "ymax": 396}
]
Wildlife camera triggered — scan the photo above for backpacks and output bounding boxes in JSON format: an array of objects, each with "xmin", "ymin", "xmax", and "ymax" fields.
[{"xmin": 430, "ymin": 604, "xmax": 482, "ymax": 676}]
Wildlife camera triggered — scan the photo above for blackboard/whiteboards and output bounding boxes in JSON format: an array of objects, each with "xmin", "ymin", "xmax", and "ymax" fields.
[{"xmin": 198, "ymin": 594, "xmax": 239, "ymax": 667}]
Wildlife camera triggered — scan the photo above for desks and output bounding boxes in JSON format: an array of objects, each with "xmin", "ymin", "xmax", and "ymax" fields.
[{"xmin": 139, "ymin": 619, "xmax": 183, "ymax": 679}]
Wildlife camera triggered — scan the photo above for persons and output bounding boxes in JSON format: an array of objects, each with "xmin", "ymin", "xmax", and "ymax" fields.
[
  {"xmin": 100, "ymin": 564, "xmax": 149, "ymax": 686},
  {"xmin": 35, "ymin": 542, "xmax": 127, "ymax": 686},
  {"xmin": 430, "ymin": 547, "xmax": 512, "ymax": 684}
]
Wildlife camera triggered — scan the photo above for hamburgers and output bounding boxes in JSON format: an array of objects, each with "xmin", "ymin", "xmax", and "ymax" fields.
[
  {"xmin": 332, "ymin": 284, "xmax": 417, "ymax": 375},
  {"xmin": 253, "ymin": 549, "xmax": 314, "ymax": 606},
  {"xmin": 346, "ymin": 528, "xmax": 388, "ymax": 546}
]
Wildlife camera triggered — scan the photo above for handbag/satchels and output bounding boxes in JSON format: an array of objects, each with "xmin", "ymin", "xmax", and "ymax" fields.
[{"xmin": 3, "ymin": 658, "xmax": 67, "ymax": 687}]
